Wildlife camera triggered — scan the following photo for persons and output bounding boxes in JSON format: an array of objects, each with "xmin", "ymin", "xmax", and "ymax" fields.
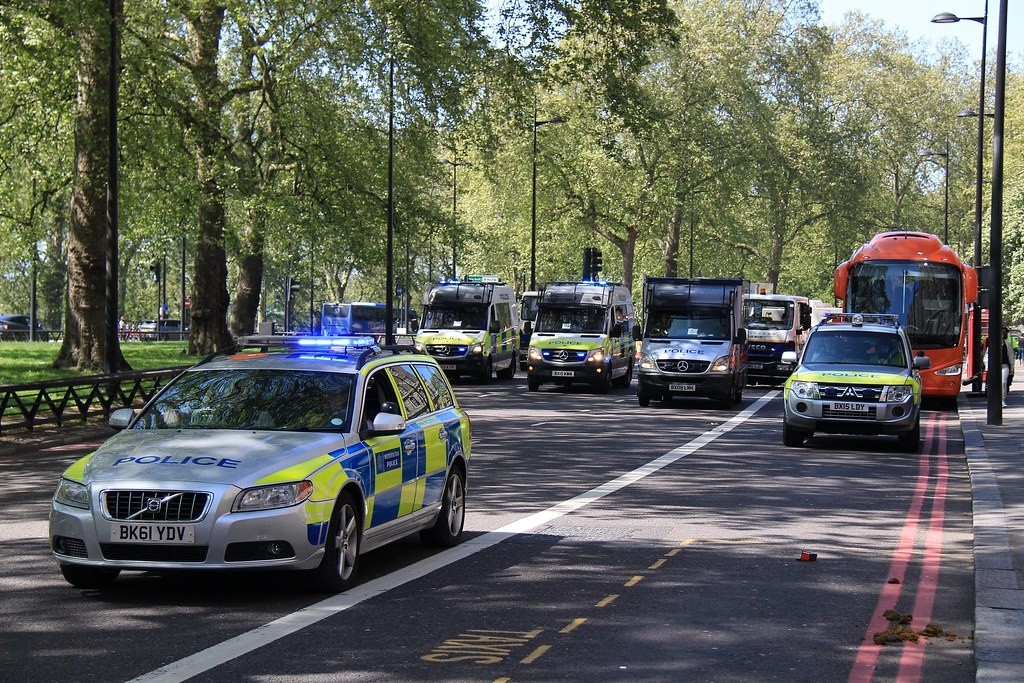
[
  {"xmin": 983, "ymin": 327, "xmax": 1014, "ymax": 408},
  {"xmin": 312, "ymin": 380, "xmax": 345, "ymax": 428},
  {"xmin": 1011, "ymin": 333, "xmax": 1024, "ymax": 360},
  {"xmin": 784, "ymin": 310, "xmax": 793, "ymax": 325}
]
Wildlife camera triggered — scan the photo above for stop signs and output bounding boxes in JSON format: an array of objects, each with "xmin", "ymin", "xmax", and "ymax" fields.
[{"xmin": 184, "ymin": 298, "xmax": 192, "ymax": 308}]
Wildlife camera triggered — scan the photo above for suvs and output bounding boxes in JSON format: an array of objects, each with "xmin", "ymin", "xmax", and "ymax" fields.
[{"xmin": 781, "ymin": 312, "xmax": 931, "ymax": 452}]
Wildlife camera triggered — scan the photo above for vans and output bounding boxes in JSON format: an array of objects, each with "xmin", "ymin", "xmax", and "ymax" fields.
[
  {"xmin": 518, "ymin": 278, "xmax": 637, "ymax": 393},
  {"xmin": 416, "ymin": 273, "xmax": 521, "ymax": 381},
  {"xmin": 631, "ymin": 276, "xmax": 817, "ymax": 408}
]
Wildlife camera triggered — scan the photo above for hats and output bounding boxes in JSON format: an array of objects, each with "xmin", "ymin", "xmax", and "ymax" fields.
[{"xmin": 319, "ymin": 375, "xmax": 352, "ymax": 392}]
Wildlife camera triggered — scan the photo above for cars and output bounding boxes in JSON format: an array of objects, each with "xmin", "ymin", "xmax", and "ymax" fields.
[
  {"xmin": 49, "ymin": 342, "xmax": 471, "ymax": 591},
  {"xmin": 138, "ymin": 318, "xmax": 192, "ymax": 340},
  {"xmin": 0, "ymin": 314, "xmax": 50, "ymax": 343}
]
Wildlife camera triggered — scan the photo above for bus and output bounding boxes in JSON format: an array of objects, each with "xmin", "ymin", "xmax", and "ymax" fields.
[
  {"xmin": 318, "ymin": 301, "xmax": 422, "ymax": 337},
  {"xmin": 833, "ymin": 230, "xmax": 978, "ymax": 407}
]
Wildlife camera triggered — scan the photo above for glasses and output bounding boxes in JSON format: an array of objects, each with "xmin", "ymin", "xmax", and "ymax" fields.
[{"xmin": 321, "ymin": 389, "xmax": 346, "ymax": 397}]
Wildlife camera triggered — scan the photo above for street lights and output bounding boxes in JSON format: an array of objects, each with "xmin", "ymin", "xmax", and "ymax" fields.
[
  {"xmin": 530, "ymin": 89, "xmax": 566, "ymax": 290},
  {"xmin": 916, "ymin": 0, "xmax": 1014, "ymax": 423}
]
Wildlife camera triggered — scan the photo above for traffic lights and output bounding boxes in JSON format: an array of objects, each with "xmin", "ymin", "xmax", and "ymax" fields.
[
  {"xmin": 287, "ymin": 275, "xmax": 302, "ymax": 302},
  {"xmin": 592, "ymin": 246, "xmax": 603, "ymax": 275},
  {"xmin": 150, "ymin": 261, "xmax": 161, "ymax": 273}
]
